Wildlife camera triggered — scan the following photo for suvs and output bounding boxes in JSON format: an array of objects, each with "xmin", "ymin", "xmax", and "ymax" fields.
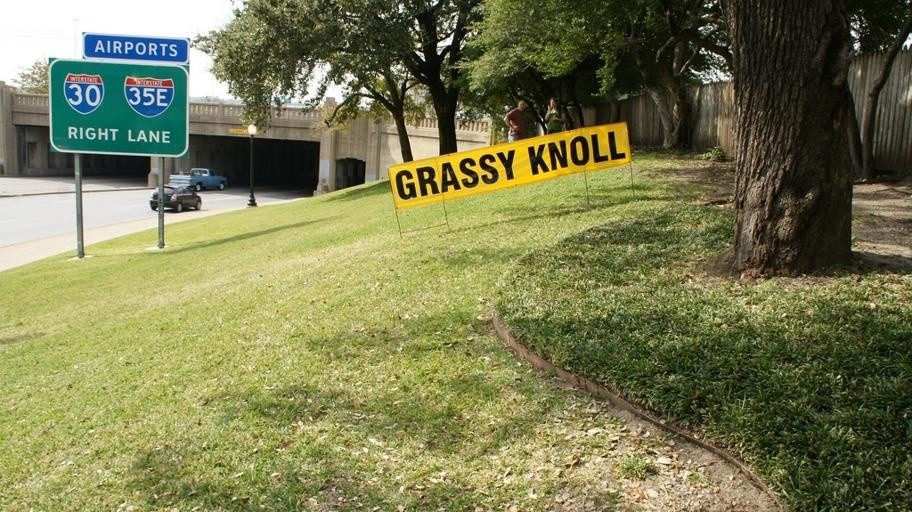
[{"xmin": 150, "ymin": 184, "xmax": 202, "ymax": 213}]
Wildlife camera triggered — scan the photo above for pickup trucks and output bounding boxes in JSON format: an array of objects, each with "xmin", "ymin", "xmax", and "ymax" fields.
[{"xmin": 170, "ymin": 167, "xmax": 229, "ymax": 192}]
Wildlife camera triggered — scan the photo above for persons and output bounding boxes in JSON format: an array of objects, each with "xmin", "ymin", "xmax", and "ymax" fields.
[{"xmin": 503, "ymin": 96, "xmax": 567, "ymax": 143}]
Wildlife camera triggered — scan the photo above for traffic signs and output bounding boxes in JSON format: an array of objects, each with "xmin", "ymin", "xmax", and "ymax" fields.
[
  {"xmin": 49, "ymin": 59, "xmax": 190, "ymax": 157},
  {"xmin": 82, "ymin": 32, "xmax": 188, "ymax": 63}
]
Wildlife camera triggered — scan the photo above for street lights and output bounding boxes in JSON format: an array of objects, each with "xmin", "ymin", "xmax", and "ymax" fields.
[{"xmin": 246, "ymin": 124, "xmax": 259, "ymax": 206}]
